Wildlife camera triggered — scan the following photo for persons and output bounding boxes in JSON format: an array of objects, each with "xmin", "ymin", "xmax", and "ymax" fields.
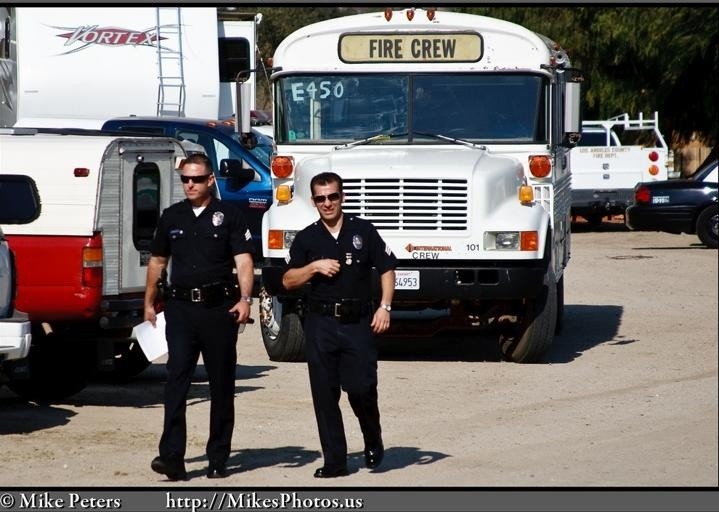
[
  {"xmin": 144, "ymin": 152, "xmax": 255, "ymax": 481},
  {"xmin": 282, "ymin": 171, "xmax": 396, "ymax": 478}
]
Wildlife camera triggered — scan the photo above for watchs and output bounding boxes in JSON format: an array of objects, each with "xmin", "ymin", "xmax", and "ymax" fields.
[
  {"xmin": 378, "ymin": 302, "xmax": 393, "ymax": 312},
  {"xmin": 238, "ymin": 295, "xmax": 253, "ymax": 305}
]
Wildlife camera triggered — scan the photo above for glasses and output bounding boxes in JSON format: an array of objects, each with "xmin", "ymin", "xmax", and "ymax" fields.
[
  {"xmin": 312, "ymin": 194, "xmax": 342, "ymax": 203},
  {"xmin": 178, "ymin": 175, "xmax": 213, "ymax": 183}
]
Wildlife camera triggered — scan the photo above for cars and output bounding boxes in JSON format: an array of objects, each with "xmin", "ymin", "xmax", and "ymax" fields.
[
  {"xmin": 623, "ymin": 157, "xmax": 719, "ymax": 252},
  {"xmin": 1, "ymin": 223, "xmax": 36, "ymax": 407}
]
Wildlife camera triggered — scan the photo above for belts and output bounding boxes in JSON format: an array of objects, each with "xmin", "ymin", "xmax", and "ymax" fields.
[
  {"xmin": 167, "ymin": 285, "xmax": 236, "ymax": 303},
  {"xmin": 305, "ymin": 302, "xmax": 373, "ymax": 316}
]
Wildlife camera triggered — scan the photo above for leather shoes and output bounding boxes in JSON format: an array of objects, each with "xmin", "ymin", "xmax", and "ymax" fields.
[
  {"xmin": 208, "ymin": 460, "xmax": 228, "ymax": 477},
  {"xmin": 314, "ymin": 461, "xmax": 349, "ymax": 478},
  {"xmin": 150, "ymin": 456, "xmax": 188, "ymax": 480},
  {"xmin": 365, "ymin": 436, "xmax": 385, "ymax": 467}
]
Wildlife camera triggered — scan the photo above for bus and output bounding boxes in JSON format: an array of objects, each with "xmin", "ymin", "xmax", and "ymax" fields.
[{"xmin": 234, "ymin": 9, "xmax": 591, "ymax": 371}]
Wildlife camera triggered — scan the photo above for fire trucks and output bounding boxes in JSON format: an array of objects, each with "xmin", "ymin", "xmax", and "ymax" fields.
[{"xmin": 564, "ymin": 111, "xmax": 673, "ymax": 231}]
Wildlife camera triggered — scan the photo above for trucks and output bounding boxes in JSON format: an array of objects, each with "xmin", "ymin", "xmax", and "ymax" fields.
[
  {"xmin": 14, "ymin": 113, "xmax": 278, "ymax": 287},
  {"xmin": 2, "ymin": 125, "xmax": 229, "ymax": 405}
]
